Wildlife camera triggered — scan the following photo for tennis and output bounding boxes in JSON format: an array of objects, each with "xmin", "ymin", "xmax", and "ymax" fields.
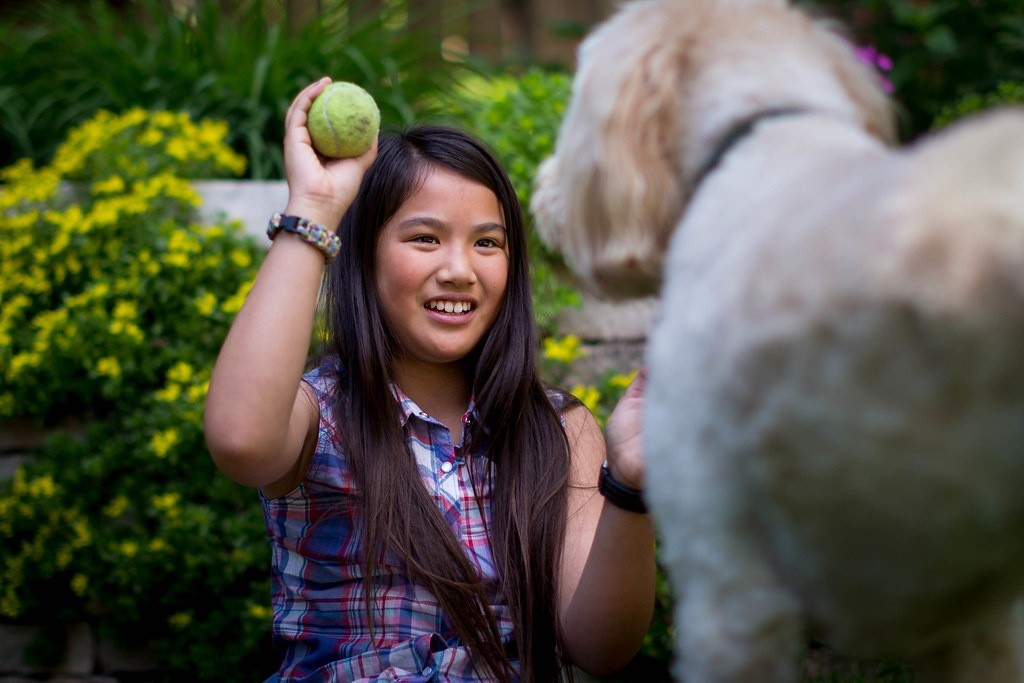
[{"xmin": 307, "ymin": 80, "xmax": 381, "ymax": 159}]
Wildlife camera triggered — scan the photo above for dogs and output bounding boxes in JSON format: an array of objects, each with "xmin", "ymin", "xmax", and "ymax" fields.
[{"xmin": 529, "ymin": 0, "xmax": 1024, "ymax": 683}]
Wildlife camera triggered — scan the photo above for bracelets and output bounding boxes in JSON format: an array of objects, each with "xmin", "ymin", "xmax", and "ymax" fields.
[
  {"xmin": 597, "ymin": 461, "xmax": 652, "ymax": 514},
  {"xmin": 266, "ymin": 213, "xmax": 342, "ymax": 264}
]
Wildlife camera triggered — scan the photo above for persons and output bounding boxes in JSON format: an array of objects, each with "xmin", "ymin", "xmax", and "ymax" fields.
[{"xmin": 203, "ymin": 77, "xmax": 656, "ymax": 683}]
